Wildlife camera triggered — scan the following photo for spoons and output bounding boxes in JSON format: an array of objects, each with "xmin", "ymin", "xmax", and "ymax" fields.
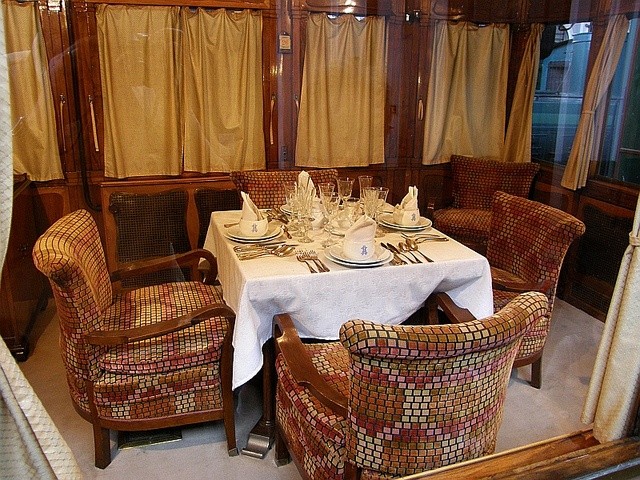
[
  {"xmin": 398, "ymin": 241, "xmax": 422, "ymax": 264},
  {"xmin": 406, "ymin": 238, "xmax": 435, "ymax": 262}
]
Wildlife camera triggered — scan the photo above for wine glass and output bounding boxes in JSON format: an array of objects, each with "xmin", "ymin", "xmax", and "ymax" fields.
[
  {"xmin": 374, "ymin": 186, "xmax": 389, "ymax": 238},
  {"xmin": 322, "ymin": 192, "xmax": 339, "ymax": 233},
  {"xmin": 359, "ymin": 175, "xmax": 374, "ymax": 200},
  {"xmin": 292, "ymin": 193, "xmax": 309, "ymax": 238},
  {"xmin": 283, "ymin": 181, "xmax": 297, "ymax": 231},
  {"xmin": 363, "ymin": 186, "xmax": 375, "ymax": 219},
  {"xmin": 317, "ymin": 181, "xmax": 336, "ymax": 199},
  {"xmin": 297, "ymin": 189, "xmax": 318, "ymax": 244},
  {"xmin": 345, "ymin": 197, "xmax": 360, "ymax": 230},
  {"xmin": 336, "ymin": 176, "xmax": 355, "ymax": 219}
]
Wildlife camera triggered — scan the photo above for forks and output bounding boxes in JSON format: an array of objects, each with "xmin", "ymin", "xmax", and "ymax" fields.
[
  {"xmin": 413, "ymin": 238, "xmax": 450, "ymax": 244},
  {"xmin": 240, "ymin": 246, "xmax": 296, "ymax": 263},
  {"xmin": 309, "ymin": 250, "xmax": 331, "ymax": 272},
  {"xmin": 294, "ymin": 249, "xmax": 316, "ymax": 274},
  {"xmin": 302, "ymin": 250, "xmax": 326, "ymax": 273},
  {"xmin": 401, "ymin": 235, "xmax": 447, "ymax": 239},
  {"xmin": 401, "ymin": 231, "xmax": 441, "ymax": 238}
]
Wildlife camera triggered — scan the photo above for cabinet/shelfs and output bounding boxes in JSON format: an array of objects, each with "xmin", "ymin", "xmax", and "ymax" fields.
[{"xmin": 1, "ymin": 182, "xmax": 46, "ymax": 362}]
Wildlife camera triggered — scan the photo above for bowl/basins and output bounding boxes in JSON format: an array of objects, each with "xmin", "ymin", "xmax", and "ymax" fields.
[
  {"xmin": 392, "ymin": 209, "xmax": 421, "ymax": 224},
  {"xmin": 339, "ymin": 239, "xmax": 378, "ymax": 260},
  {"xmin": 236, "ymin": 220, "xmax": 270, "ymax": 236}
]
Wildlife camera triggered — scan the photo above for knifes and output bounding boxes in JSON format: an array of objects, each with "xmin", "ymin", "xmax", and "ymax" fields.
[
  {"xmin": 386, "ymin": 242, "xmax": 416, "ymax": 265},
  {"xmin": 381, "ymin": 242, "xmax": 401, "ymax": 265},
  {"xmin": 235, "ymin": 239, "xmax": 288, "ymax": 248}
]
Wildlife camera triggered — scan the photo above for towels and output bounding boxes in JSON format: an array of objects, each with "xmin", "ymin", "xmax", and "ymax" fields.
[
  {"xmin": 345, "ymin": 215, "xmax": 377, "ymax": 242},
  {"xmin": 397, "ymin": 186, "xmax": 419, "ymax": 209},
  {"xmin": 239, "ymin": 191, "xmax": 265, "ymax": 221},
  {"xmin": 298, "ymin": 171, "xmax": 317, "ymax": 200}
]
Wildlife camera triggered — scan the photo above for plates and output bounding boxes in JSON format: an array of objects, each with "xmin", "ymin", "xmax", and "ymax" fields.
[
  {"xmin": 376, "ymin": 217, "xmax": 433, "ymax": 232},
  {"xmin": 279, "ymin": 207, "xmax": 287, "ymax": 215},
  {"xmin": 379, "ymin": 214, "xmax": 431, "ymax": 228},
  {"xmin": 226, "ymin": 222, "xmax": 280, "ymax": 240},
  {"xmin": 329, "ymin": 242, "xmax": 389, "ymax": 263},
  {"xmin": 223, "ymin": 228, "xmax": 285, "ymax": 243},
  {"xmin": 282, "ymin": 204, "xmax": 293, "ymax": 213},
  {"xmin": 322, "ymin": 246, "xmax": 397, "ymax": 269}
]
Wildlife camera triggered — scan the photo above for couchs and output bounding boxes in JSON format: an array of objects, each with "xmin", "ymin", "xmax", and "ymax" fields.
[
  {"xmin": 432, "ymin": 156, "xmax": 540, "ymax": 250},
  {"xmin": 231, "ymin": 168, "xmax": 340, "ymax": 209},
  {"xmin": 273, "ymin": 290, "xmax": 548, "ymax": 479},
  {"xmin": 487, "ymin": 190, "xmax": 585, "ymax": 387},
  {"xmin": 33, "ymin": 210, "xmax": 240, "ymax": 470}
]
